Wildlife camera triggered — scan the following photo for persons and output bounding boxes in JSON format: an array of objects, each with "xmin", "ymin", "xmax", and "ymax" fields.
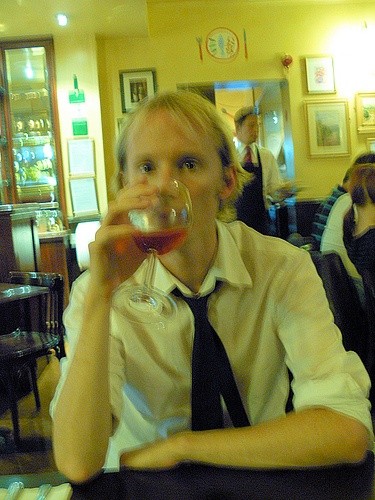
[{"xmin": 49, "ymin": 90, "xmax": 375, "ymax": 483}]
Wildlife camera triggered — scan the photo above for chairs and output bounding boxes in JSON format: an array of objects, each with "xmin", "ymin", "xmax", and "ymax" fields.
[
  {"xmin": 276, "ymin": 200, "xmax": 368, "ymax": 355},
  {"xmin": 0, "ymin": 272, "xmax": 66, "ymax": 446}
]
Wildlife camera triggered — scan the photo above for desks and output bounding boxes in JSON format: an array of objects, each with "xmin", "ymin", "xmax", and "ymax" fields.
[
  {"xmin": 0, "ymin": 282, "xmax": 50, "ymax": 408},
  {"xmin": 0, "ymin": 441, "xmax": 375, "ymax": 500}
]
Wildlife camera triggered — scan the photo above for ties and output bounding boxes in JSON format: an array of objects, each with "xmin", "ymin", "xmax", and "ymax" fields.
[
  {"xmin": 172, "ymin": 281, "xmax": 254, "ymax": 430},
  {"xmin": 242, "ymin": 145, "xmax": 255, "ymax": 162}
]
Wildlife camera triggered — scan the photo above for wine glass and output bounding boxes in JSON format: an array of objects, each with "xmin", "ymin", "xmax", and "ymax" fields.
[{"xmin": 110, "ymin": 177, "xmax": 193, "ymax": 326}]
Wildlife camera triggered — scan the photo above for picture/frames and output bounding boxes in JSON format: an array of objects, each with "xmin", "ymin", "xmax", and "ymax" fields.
[
  {"xmin": 304, "ymin": 100, "xmax": 352, "ymax": 159},
  {"xmin": 119, "ymin": 68, "xmax": 157, "ymax": 113},
  {"xmin": 355, "ymin": 92, "xmax": 375, "ymax": 133},
  {"xmin": 367, "ymin": 138, "xmax": 375, "ymax": 154},
  {"xmin": 305, "ymin": 57, "xmax": 336, "ymax": 94}
]
